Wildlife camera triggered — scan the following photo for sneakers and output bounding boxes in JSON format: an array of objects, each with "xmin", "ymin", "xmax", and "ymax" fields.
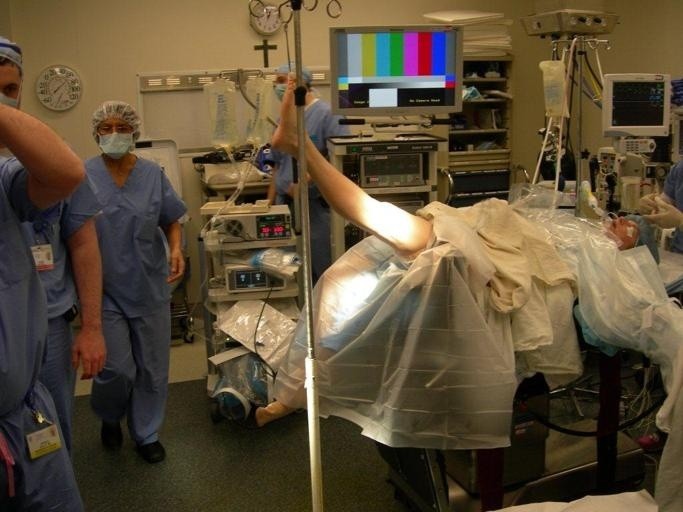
[
  {"xmin": 101, "ymin": 420, "xmax": 123, "ymax": 452},
  {"xmin": 139, "ymin": 441, "xmax": 165, "ymax": 465},
  {"xmin": 637, "ymin": 433, "xmax": 666, "ymax": 453}
]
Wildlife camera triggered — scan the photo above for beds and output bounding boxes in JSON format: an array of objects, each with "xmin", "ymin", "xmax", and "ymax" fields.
[{"xmin": 268, "ymin": 196, "xmax": 683, "ymax": 508}]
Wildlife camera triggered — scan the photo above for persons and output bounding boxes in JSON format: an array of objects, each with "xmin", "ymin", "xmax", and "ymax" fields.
[
  {"xmin": 635, "ymin": 158, "xmax": 681, "ymax": 255},
  {"xmin": 0, "ymin": 37, "xmax": 107, "ymax": 457},
  {"xmin": 82, "ymin": 100, "xmax": 188, "ymax": 463},
  {"xmin": 256, "ymin": 74, "xmax": 640, "ymax": 255},
  {"xmin": 0, "ymin": 105, "xmax": 86, "ymax": 512},
  {"xmin": 267, "ymin": 62, "xmax": 351, "ymax": 287}
]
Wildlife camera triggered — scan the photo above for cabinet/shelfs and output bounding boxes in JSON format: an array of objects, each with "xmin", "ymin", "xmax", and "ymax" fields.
[
  {"xmin": 205, "ymin": 182, "xmax": 305, "ymax": 406},
  {"xmin": 437, "ymin": 57, "xmax": 514, "ymax": 205}
]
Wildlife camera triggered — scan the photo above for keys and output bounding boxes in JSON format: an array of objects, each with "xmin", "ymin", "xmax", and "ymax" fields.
[{"xmin": 36, "ymin": 410, "xmax": 54, "ymax": 425}]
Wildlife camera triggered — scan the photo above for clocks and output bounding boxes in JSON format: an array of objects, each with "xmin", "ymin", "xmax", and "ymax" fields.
[
  {"xmin": 35, "ymin": 64, "xmax": 82, "ymax": 111},
  {"xmin": 250, "ymin": 2, "xmax": 283, "ymax": 35}
]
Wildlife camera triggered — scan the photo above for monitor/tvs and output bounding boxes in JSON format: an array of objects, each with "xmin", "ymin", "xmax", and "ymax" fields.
[
  {"xmin": 601, "ymin": 73, "xmax": 671, "ymax": 137},
  {"xmin": 328, "ymin": 23, "xmax": 464, "ymax": 117}
]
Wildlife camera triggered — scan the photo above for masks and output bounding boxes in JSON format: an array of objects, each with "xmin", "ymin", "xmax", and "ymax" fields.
[
  {"xmin": 96, "ymin": 132, "xmax": 134, "ymax": 160},
  {"xmin": 274, "ymin": 84, "xmax": 286, "ymax": 101}
]
